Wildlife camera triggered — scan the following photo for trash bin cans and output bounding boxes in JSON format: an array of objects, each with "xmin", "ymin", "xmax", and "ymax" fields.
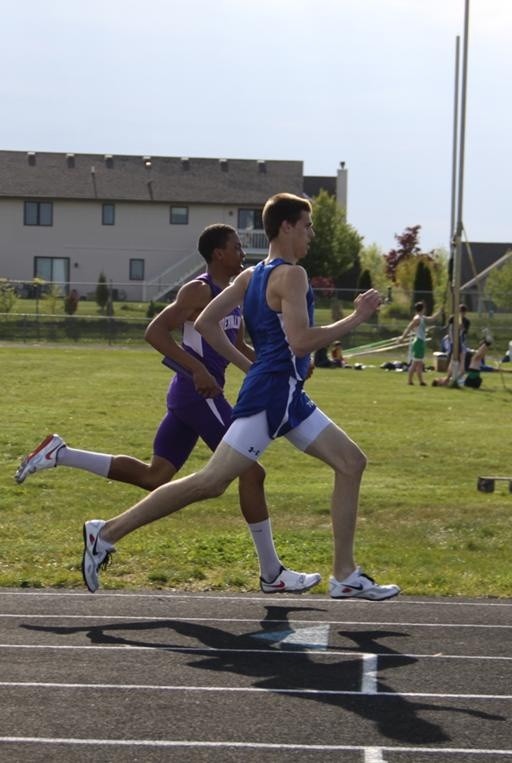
[
  {"xmin": 112, "ymin": 289, "xmax": 119, "ymax": 301},
  {"xmin": 433, "ymin": 352, "xmax": 449, "ymax": 372}
]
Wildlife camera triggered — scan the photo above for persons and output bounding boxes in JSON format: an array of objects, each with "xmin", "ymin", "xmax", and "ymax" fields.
[
  {"xmin": 80, "ymin": 192, "xmax": 401, "ymax": 602},
  {"xmin": 397, "ymin": 301, "xmax": 512, "ymax": 388},
  {"xmin": 16, "ymin": 222, "xmax": 321, "ymax": 595}
]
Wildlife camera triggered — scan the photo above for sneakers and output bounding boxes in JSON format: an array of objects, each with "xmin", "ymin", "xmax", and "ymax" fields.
[
  {"xmin": 328, "ymin": 568, "xmax": 400, "ymax": 602},
  {"xmin": 15, "ymin": 432, "xmax": 66, "ymax": 483},
  {"xmin": 258, "ymin": 565, "xmax": 322, "ymax": 594},
  {"xmin": 82, "ymin": 518, "xmax": 117, "ymax": 592}
]
